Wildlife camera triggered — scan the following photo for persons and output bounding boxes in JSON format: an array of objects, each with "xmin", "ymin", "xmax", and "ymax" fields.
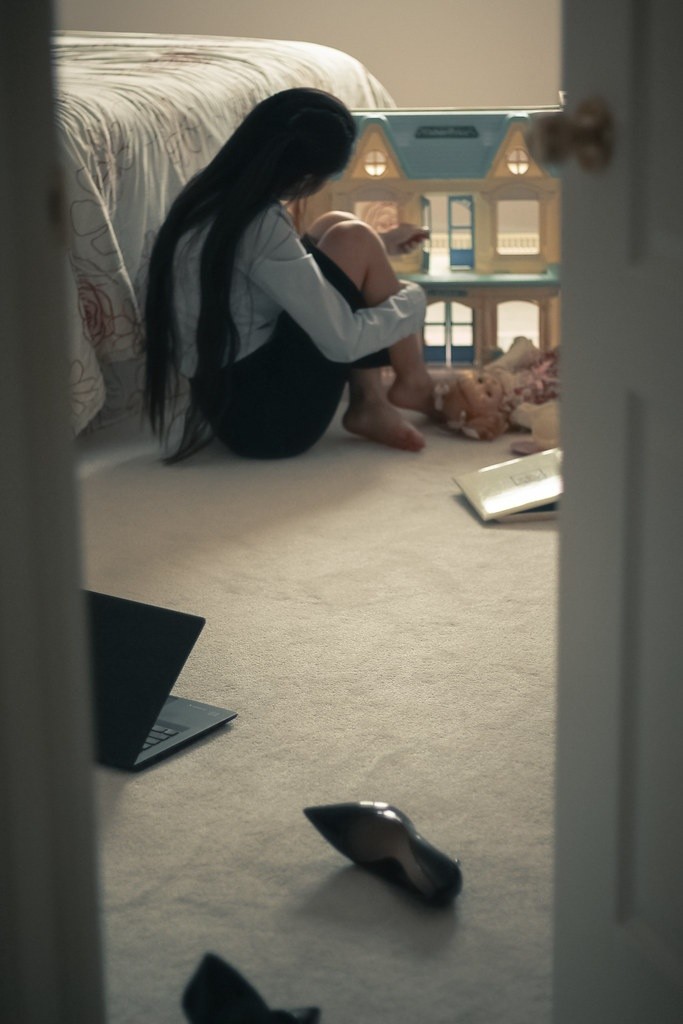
[{"xmin": 145, "ymin": 86, "xmax": 440, "ymax": 461}]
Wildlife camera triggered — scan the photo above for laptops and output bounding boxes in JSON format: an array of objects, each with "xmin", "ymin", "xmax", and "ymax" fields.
[{"xmin": 81, "ymin": 589, "xmax": 239, "ymax": 772}]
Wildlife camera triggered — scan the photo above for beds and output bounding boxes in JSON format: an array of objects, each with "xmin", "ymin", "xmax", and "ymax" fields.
[{"xmin": 52, "ymin": 30, "xmax": 398, "ymax": 474}]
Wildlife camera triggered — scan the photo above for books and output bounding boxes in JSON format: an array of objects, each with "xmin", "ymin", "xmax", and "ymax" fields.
[{"xmin": 454, "ymin": 446, "xmax": 563, "ymax": 523}]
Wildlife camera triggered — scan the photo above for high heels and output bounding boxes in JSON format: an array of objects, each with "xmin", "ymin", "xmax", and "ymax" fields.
[
  {"xmin": 181, "ymin": 947, "xmax": 324, "ymax": 1023},
  {"xmin": 301, "ymin": 798, "xmax": 467, "ymax": 908}
]
